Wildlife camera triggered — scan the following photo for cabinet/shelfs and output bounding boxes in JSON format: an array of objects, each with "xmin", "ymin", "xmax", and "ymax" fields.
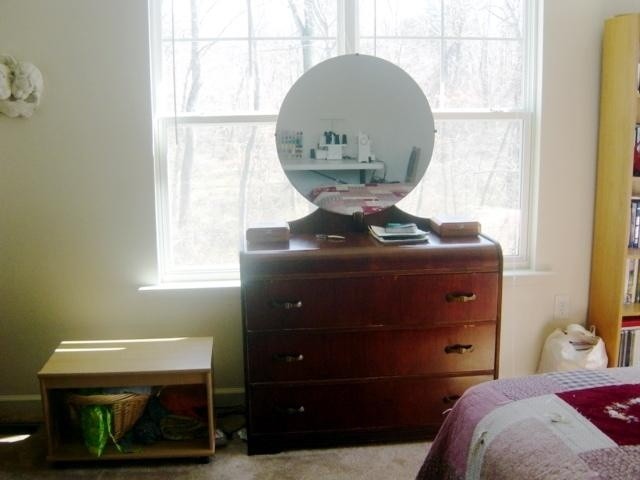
[
  {"xmin": 585, "ymin": 13, "xmax": 639, "ymax": 370},
  {"xmin": 237, "ymin": 234, "xmax": 504, "ymax": 456}
]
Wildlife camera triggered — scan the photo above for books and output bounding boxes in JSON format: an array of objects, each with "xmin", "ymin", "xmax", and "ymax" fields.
[
  {"xmin": 246, "ymin": 221, "xmax": 290, "ymax": 241},
  {"xmin": 618, "ymin": 125, "xmax": 640, "ymax": 368},
  {"xmin": 431, "ymin": 213, "xmax": 481, "ymax": 237},
  {"xmin": 368, "ymin": 222, "xmax": 431, "ymax": 243}
]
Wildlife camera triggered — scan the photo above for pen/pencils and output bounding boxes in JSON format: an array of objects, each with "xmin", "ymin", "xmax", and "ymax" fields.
[{"xmin": 315, "ymin": 234, "xmax": 346, "ymax": 240}]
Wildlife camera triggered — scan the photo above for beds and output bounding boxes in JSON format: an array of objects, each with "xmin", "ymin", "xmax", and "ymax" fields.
[
  {"xmin": 442, "ymin": 356, "xmax": 640, "ymax": 479},
  {"xmin": 302, "ymin": 183, "xmax": 418, "ymax": 216}
]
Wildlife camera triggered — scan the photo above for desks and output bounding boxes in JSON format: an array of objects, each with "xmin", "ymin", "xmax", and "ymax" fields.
[
  {"xmin": 281, "ymin": 160, "xmax": 386, "ymax": 183},
  {"xmin": 37, "ymin": 338, "xmax": 215, "ymax": 463}
]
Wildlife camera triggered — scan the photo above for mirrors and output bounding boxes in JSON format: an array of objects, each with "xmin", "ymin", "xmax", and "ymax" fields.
[{"xmin": 274, "ymin": 52, "xmax": 438, "ymax": 218}]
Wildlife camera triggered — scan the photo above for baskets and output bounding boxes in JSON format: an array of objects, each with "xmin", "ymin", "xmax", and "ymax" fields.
[{"xmin": 67, "ymin": 391, "xmax": 150, "ymax": 438}]
